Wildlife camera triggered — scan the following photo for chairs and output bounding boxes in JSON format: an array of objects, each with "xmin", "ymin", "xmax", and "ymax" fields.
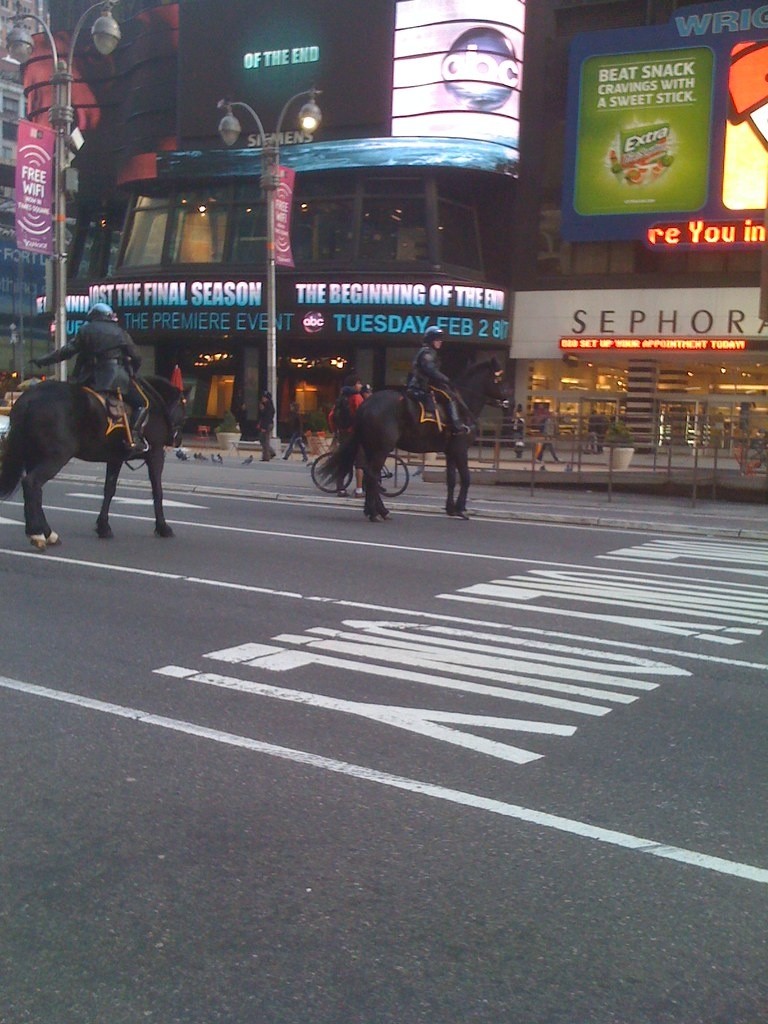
[
  {"xmin": 315, "ymin": 431, "xmax": 330, "ymax": 454},
  {"xmin": 306, "ymin": 430, "xmax": 321, "ymax": 457},
  {"xmin": 191, "ymin": 425, "xmax": 212, "ymax": 451}
]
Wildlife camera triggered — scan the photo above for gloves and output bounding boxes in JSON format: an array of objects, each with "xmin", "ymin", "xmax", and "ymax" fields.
[{"xmin": 29, "ymin": 359, "xmax": 45, "ymax": 368}]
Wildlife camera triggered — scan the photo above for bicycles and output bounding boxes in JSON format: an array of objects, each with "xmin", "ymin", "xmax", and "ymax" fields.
[{"xmin": 311, "ymin": 436, "xmax": 410, "ymax": 497}]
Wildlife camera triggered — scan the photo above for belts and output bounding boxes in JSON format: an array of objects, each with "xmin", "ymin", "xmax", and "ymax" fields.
[{"xmin": 83, "ymin": 359, "xmax": 123, "ymax": 365}]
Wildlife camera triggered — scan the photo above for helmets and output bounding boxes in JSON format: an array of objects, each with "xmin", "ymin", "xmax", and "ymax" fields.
[
  {"xmin": 361, "ymin": 385, "xmax": 373, "ymax": 391},
  {"xmin": 423, "ymin": 326, "xmax": 443, "ymax": 343},
  {"xmin": 85, "ymin": 303, "xmax": 117, "ymax": 322}
]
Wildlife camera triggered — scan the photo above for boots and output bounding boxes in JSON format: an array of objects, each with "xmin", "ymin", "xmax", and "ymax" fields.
[{"xmin": 122, "ymin": 407, "xmax": 147, "ymax": 450}]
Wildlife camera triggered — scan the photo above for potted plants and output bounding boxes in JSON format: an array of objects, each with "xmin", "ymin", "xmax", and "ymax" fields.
[
  {"xmin": 602, "ymin": 421, "xmax": 636, "ymax": 470},
  {"xmin": 216, "ymin": 411, "xmax": 241, "ymax": 451}
]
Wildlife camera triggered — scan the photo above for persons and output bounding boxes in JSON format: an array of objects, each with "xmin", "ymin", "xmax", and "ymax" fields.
[
  {"xmin": 327, "ymin": 374, "xmax": 374, "ymax": 498},
  {"xmin": 254, "ymin": 390, "xmax": 277, "ymax": 463},
  {"xmin": 281, "ymin": 401, "xmax": 310, "ymax": 462},
  {"xmin": 404, "ymin": 326, "xmax": 470, "ymax": 436},
  {"xmin": 584, "ymin": 409, "xmax": 611, "ymax": 455},
  {"xmin": 27, "ymin": 303, "xmax": 152, "ymax": 451},
  {"xmin": 509, "ymin": 410, "xmax": 525, "ymax": 459},
  {"xmin": 535, "ymin": 410, "xmax": 565, "ymax": 465}
]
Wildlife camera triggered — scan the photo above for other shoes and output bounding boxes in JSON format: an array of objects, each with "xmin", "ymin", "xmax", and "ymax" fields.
[
  {"xmin": 355, "ymin": 490, "xmax": 366, "ymax": 497},
  {"xmin": 337, "ymin": 490, "xmax": 350, "ymax": 497}
]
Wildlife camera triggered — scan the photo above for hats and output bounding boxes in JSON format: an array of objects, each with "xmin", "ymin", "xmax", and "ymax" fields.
[{"xmin": 263, "ymin": 391, "xmax": 272, "ymax": 400}]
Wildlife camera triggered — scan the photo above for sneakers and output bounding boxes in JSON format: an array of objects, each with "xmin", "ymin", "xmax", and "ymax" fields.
[{"xmin": 452, "ymin": 424, "xmax": 470, "ymax": 436}]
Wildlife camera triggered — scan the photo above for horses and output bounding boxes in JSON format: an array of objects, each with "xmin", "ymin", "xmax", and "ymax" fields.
[
  {"xmin": 312, "ymin": 357, "xmax": 510, "ymax": 522},
  {"xmin": 0, "ymin": 374, "xmax": 193, "ymax": 549}
]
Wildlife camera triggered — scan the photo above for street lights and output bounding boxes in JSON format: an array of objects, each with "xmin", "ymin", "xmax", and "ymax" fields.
[
  {"xmin": 220, "ymin": 82, "xmax": 324, "ymax": 449},
  {"xmin": 4, "ymin": 0, "xmax": 121, "ymax": 381}
]
